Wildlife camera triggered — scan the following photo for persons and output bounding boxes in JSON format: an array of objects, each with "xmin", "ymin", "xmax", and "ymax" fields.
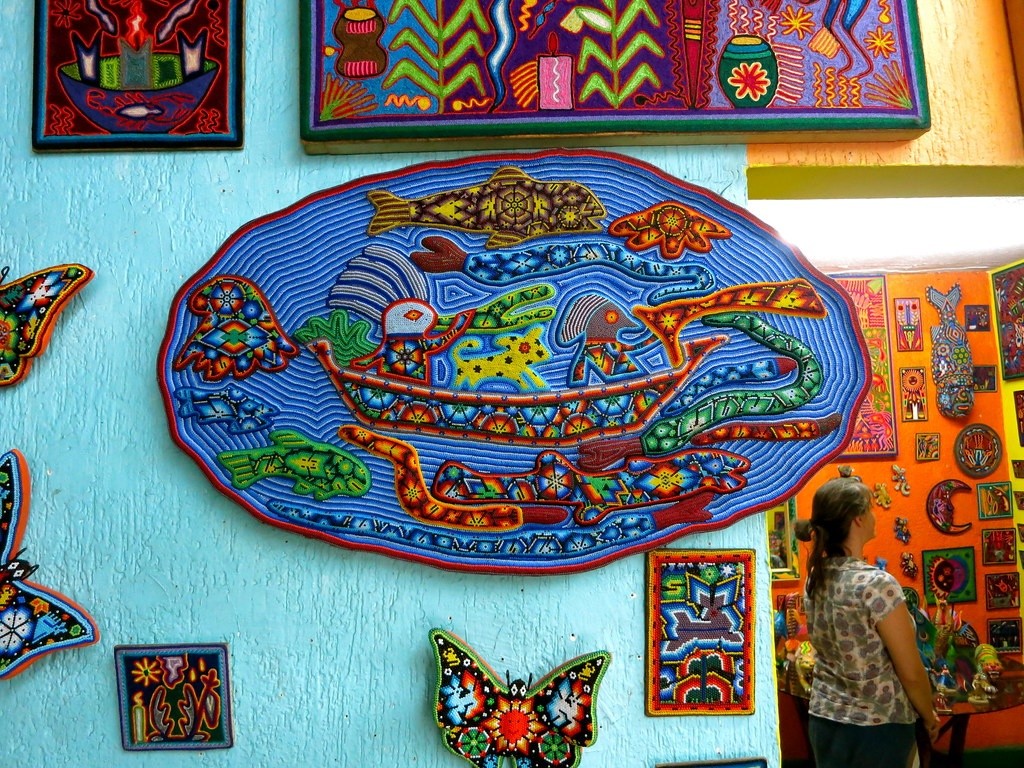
[{"xmin": 795, "ymin": 475, "xmax": 940, "ymax": 768}]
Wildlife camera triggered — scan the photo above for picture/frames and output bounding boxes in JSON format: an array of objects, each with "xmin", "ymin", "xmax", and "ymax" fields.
[
  {"xmin": 32, "ymin": 0, "xmax": 245, "ymax": 154},
  {"xmin": 298, "ymin": 0, "xmax": 930, "ymax": 140}
]
